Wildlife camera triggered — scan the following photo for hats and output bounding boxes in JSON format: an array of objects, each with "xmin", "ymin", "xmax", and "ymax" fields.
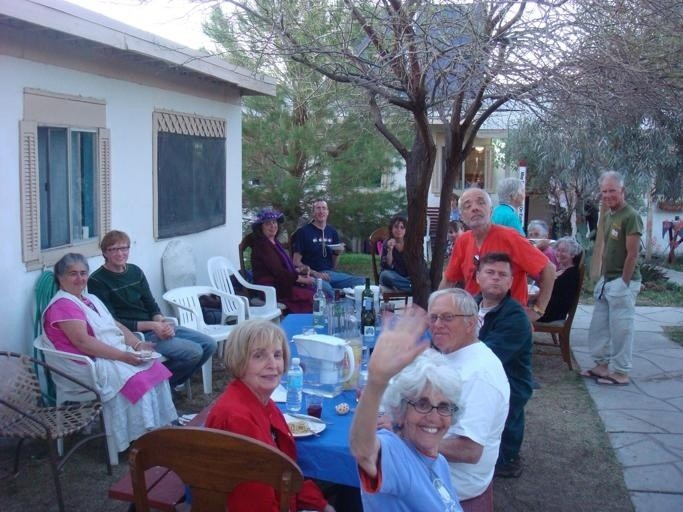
[{"xmin": 251, "ymin": 207, "xmax": 285, "ymax": 225}]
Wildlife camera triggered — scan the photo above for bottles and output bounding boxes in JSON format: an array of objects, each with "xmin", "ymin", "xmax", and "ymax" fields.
[
  {"xmin": 285, "ymin": 358, "xmax": 303, "ymax": 412},
  {"xmin": 312, "ymin": 277, "xmax": 327, "ymax": 329},
  {"xmin": 376, "ymin": 284, "xmax": 385, "ymax": 308},
  {"xmin": 333, "ymin": 290, "xmax": 344, "ymax": 329},
  {"xmin": 360, "ymin": 276, "xmax": 374, "ymax": 386}
]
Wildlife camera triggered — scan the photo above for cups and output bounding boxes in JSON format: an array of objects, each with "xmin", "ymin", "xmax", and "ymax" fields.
[
  {"xmin": 304, "ymin": 393, "xmax": 322, "ymax": 418},
  {"xmin": 140, "ymin": 340, "xmax": 152, "ymax": 356},
  {"xmin": 352, "ymin": 286, "xmax": 379, "ymax": 322},
  {"xmin": 72, "ymin": 225, "xmax": 89, "ymax": 242},
  {"xmin": 379, "ymin": 302, "xmax": 395, "ymax": 323}
]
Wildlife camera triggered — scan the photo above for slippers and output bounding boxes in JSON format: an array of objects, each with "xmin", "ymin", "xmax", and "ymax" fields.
[
  {"xmin": 578, "ymin": 368, "xmax": 608, "ymax": 378},
  {"xmin": 595, "ymin": 374, "xmax": 630, "ymax": 386}
]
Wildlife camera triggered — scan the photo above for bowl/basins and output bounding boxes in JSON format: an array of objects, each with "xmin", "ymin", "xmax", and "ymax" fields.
[{"xmin": 527, "ymin": 285, "xmax": 539, "ymax": 302}]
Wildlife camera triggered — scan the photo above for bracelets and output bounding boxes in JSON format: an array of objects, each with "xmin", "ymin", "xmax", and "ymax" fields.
[{"xmin": 133, "ymin": 341, "xmax": 142, "ymax": 351}]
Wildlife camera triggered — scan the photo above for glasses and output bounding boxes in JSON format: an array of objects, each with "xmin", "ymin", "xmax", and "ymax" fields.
[
  {"xmin": 429, "ymin": 311, "xmax": 475, "ymax": 323},
  {"xmin": 64, "ymin": 270, "xmax": 89, "ymax": 277},
  {"xmin": 106, "ymin": 245, "xmax": 130, "ymax": 253},
  {"xmin": 402, "ymin": 396, "xmax": 458, "ymax": 418}
]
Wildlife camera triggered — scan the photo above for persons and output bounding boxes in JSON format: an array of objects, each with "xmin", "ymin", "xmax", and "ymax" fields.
[
  {"xmin": 439, "ymin": 188, "xmax": 557, "ymax": 322},
  {"xmin": 87, "ymin": 230, "xmax": 218, "ymax": 387},
  {"xmin": 374, "ymin": 288, "xmax": 510, "ymax": 512},
  {"xmin": 193, "ymin": 319, "xmax": 337, "ymax": 512},
  {"xmin": 576, "ymin": 170, "xmax": 643, "ymax": 386},
  {"xmin": 238, "ymin": 199, "xmax": 367, "ymax": 315},
  {"xmin": 470, "ymin": 253, "xmax": 534, "ymax": 479},
  {"xmin": 40, "ymin": 252, "xmax": 179, "ymax": 468},
  {"xmin": 378, "ymin": 216, "xmax": 413, "ymax": 289},
  {"xmin": 446, "ymin": 177, "xmax": 582, "ymax": 323},
  {"xmin": 348, "ymin": 303, "xmax": 466, "ymax": 512}
]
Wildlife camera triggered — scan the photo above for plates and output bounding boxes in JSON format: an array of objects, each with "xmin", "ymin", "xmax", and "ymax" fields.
[
  {"xmin": 126, "ymin": 352, "xmax": 161, "ymax": 360},
  {"xmin": 179, "ymin": 414, "xmax": 202, "ymax": 426},
  {"xmin": 280, "ymin": 411, "xmax": 325, "ymax": 438},
  {"xmin": 526, "ymin": 238, "xmax": 557, "ymax": 246},
  {"xmin": 326, "ymin": 244, "xmax": 345, "ymax": 249}
]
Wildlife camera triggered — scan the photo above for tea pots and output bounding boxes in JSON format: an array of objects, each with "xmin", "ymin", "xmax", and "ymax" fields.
[
  {"xmin": 341, "ymin": 337, "xmax": 371, "ymax": 392},
  {"xmin": 293, "ymin": 332, "xmax": 355, "ymax": 398}
]
